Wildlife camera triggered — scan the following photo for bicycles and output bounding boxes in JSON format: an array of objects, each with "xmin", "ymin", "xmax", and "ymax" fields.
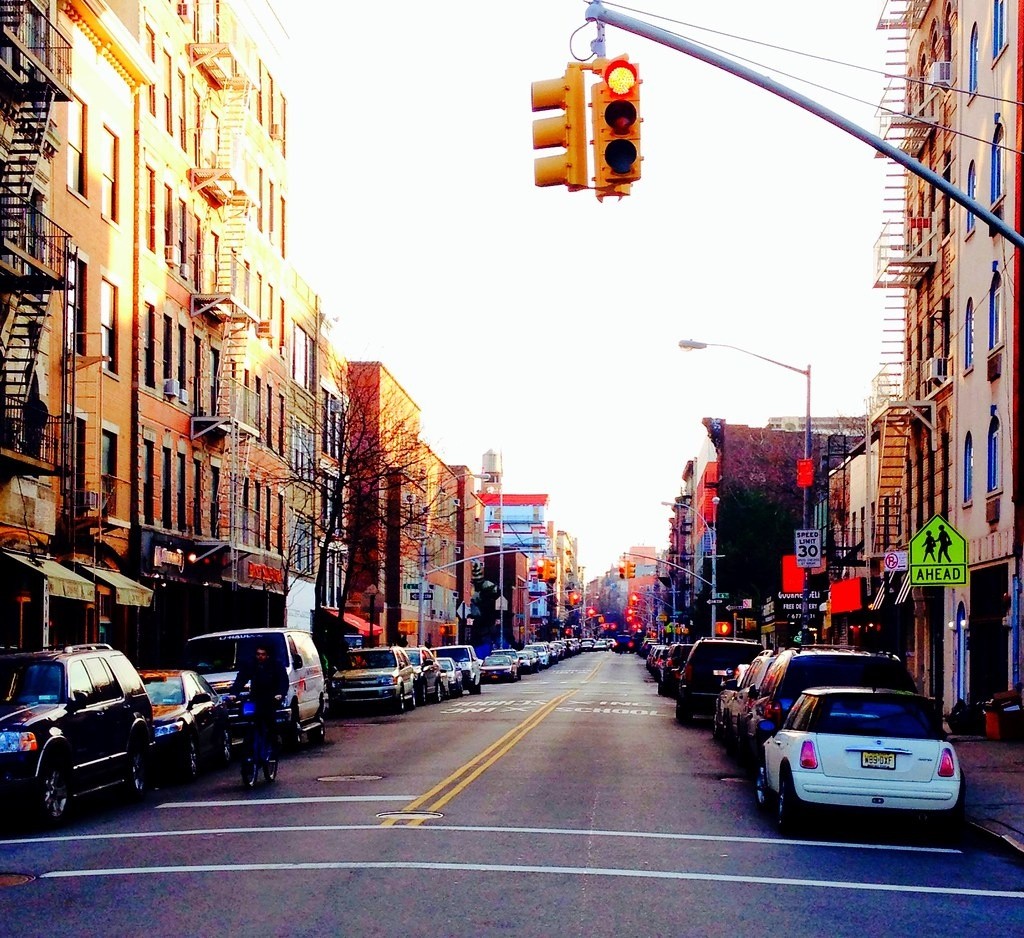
[{"xmin": 226, "ymin": 694, "xmax": 279, "ymax": 787}]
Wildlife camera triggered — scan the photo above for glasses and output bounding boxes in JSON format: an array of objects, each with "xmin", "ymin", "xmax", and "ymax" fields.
[{"xmin": 256, "ymin": 652, "xmax": 266, "ymax": 656}]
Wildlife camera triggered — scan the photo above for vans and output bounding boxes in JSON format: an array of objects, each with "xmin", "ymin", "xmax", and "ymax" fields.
[
  {"xmin": 181, "ymin": 627, "xmax": 326, "ymax": 757},
  {"xmin": 429, "ymin": 644, "xmax": 480, "ymax": 694}
]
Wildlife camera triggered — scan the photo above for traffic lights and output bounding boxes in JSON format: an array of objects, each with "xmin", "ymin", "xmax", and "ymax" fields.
[
  {"xmin": 589, "ymin": 53, "xmax": 645, "ymax": 203},
  {"xmin": 531, "ymin": 62, "xmax": 589, "ymax": 192},
  {"xmin": 627, "ymin": 558, "xmax": 636, "ymax": 579},
  {"xmin": 619, "ymin": 560, "xmax": 626, "ymax": 579},
  {"xmin": 570, "ymin": 591, "xmax": 578, "ymax": 606},
  {"xmin": 629, "ymin": 593, "xmax": 637, "ymax": 606}
]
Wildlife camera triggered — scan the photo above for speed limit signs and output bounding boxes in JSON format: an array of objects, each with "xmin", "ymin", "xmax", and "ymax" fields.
[{"xmin": 794, "ymin": 528, "xmax": 822, "ymax": 568}]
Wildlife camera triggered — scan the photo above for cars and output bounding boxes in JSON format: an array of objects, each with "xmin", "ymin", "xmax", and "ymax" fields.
[
  {"xmin": 641, "ymin": 638, "xmax": 695, "ymax": 686},
  {"xmin": 328, "ymin": 646, "xmax": 416, "ymax": 713},
  {"xmin": 435, "ymin": 657, "xmax": 463, "ymax": 697},
  {"xmin": 755, "ymin": 685, "xmax": 961, "ymax": 836},
  {"xmin": 136, "ymin": 669, "xmax": 232, "ymax": 779},
  {"xmin": 480, "ymin": 638, "xmax": 615, "ymax": 683}
]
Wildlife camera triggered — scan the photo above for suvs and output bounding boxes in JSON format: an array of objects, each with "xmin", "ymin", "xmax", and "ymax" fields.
[
  {"xmin": 713, "ymin": 644, "xmax": 920, "ymax": 767},
  {"xmin": 614, "ymin": 635, "xmax": 632, "ymax": 653},
  {"xmin": 674, "ymin": 638, "xmax": 764, "ymax": 722},
  {"xmin": 403, "ymin": 645, "xmax": 443, "ymax": 706},
  {"xmin": 0, "ymin": 643, "xmax": 154, "ymax": 827}
]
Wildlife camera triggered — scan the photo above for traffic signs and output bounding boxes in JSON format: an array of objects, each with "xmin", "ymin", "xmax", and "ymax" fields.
[
  {"xmin": 725, "ymin": 604, "xmax": 743, "ymax": 612},
  {"xmin": 706, "ymin": 599, "xmax": 723, "ymax": 605}
]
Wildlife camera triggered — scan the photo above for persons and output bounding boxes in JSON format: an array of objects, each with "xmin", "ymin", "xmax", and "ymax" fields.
[{"xmin": 229, "ymin": 638, "xmax": 290, "ymax": 766}]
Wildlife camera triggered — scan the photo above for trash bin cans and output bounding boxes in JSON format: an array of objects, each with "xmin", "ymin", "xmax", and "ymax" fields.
[{"xmin": 984, "ymin": 710, "xmax": 1024, "ymax": 742}]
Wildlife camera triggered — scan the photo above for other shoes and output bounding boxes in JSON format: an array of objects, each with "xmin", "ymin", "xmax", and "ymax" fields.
[{"xmin": 265, "ymin": 749, "xmax": 273, "ymax": 762}]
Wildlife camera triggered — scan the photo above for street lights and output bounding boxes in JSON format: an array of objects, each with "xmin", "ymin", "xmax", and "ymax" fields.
[
  {"xmin": 499, "ymin": 533, "xmax": 547, "ymax": 651},
  {"xmin": 679, "ymin": 339, "xmax": 812, "ymax": 644},
  {"xmin": 662, "ymin": 497, "xmax": 717, "ymax": 637},
  {"xmin": 420, "ymin": 473, "xmax": 491, "ymax": 646},
  {"xmin": 583, "ymin": 595, "xmax": 599, "ymax": 637}
]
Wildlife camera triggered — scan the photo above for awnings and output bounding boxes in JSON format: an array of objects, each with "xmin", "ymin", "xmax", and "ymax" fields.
[
  {"xmin": 5, "ymin": 552, "xmax": 95, "ymax": 602},
  {"xmin": 320, "ymin": 607, "xmax": 384, "ymax": 638},
  {"xmin": 75, "ymin": 561, "xmax": 153, "ymax": 609}
]
